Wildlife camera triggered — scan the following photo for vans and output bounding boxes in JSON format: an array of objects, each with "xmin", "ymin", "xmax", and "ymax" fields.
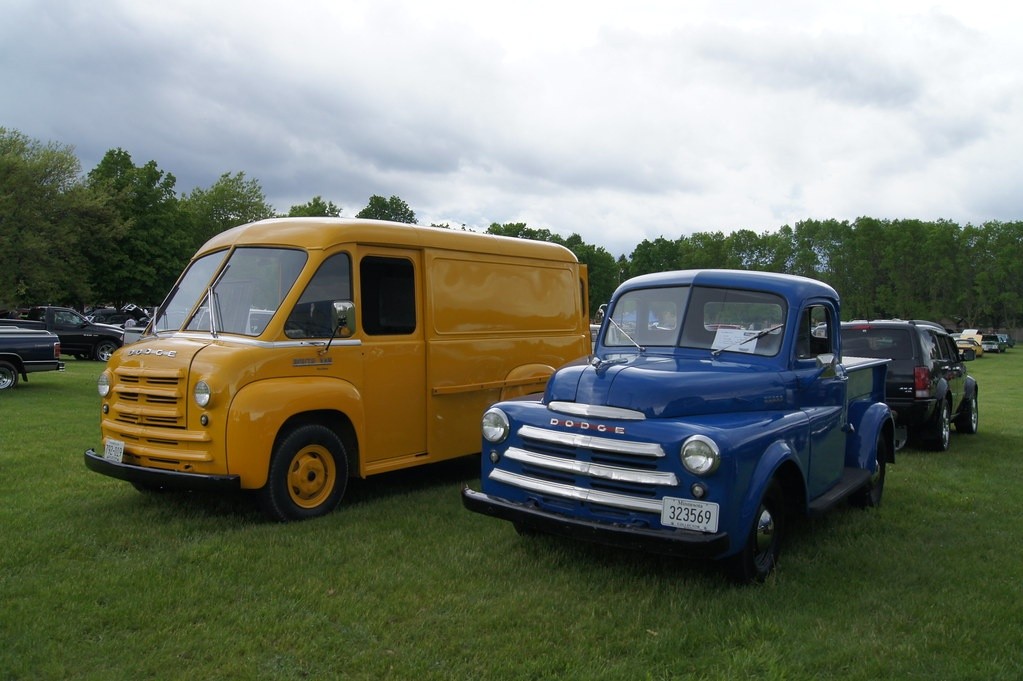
[
  {"xmin": 196, "ymin": 309, "xmax": 276, "ymax": 337},
  {"xmin": 84, "ymin": 216, "xmax": 592, "ymax": 523}
]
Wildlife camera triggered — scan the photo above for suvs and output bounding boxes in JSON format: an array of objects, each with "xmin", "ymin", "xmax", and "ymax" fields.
[{"xmin": 812, "ymin": 318, "xmax": 978, "ymax": 453}]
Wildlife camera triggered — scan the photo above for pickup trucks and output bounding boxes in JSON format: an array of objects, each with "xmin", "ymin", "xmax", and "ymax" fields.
[
  {"xmin": 0, "ymin": 302, "xmax": 152, "ymax": 390},
  {"xmin": 461, "ymin": 269, "xmax": 895, "ymax": 587}
]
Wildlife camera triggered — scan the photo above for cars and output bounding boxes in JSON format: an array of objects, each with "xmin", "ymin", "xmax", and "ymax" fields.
[{"xmin": 590, "ymin": 323, "xmax": 1015, "ymax": 360}]
